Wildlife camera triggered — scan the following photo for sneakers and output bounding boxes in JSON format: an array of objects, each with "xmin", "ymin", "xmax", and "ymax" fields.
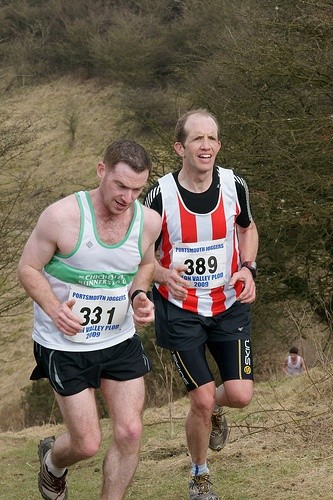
[
  {"xmin": 37, "ymin": 436, "xmax": 68, "ymax": 500},
  {"xmin": 188, "ymin": 468, "xmax": 218, "ymax": 500},
  {"xmin": 209, "ymin": 405, "xmax": 229, "ymax": 452}
]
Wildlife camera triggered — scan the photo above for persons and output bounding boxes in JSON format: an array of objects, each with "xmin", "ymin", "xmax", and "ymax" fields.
[
  {"xmin": 18, "ymin": 140, "xmax": 162, "ymax": 500},
  {"xmin": 143, "ymin": 110, "xmax": 259, "ymax": 500},
  {"xmin": 281, "ymin": 347, "xmax": 308, "ymax": 379}
]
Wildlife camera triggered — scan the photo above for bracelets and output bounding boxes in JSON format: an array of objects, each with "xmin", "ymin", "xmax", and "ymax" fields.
[
  {"xmin": 131, "ymin": 289, "xmax": 146, "ymax": 302},
  {"xmin": 241, "ymin": 260, "xmax": 259, "ymax": 278}
]
